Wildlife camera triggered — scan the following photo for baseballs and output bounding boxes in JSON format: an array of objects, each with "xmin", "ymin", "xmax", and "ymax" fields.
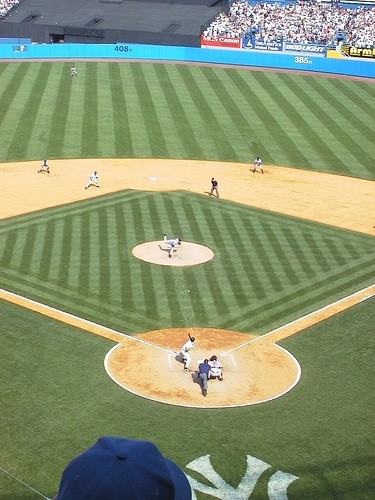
[{"xmin": 187, "ymin": 289, "xmax": 190, "ymax": 293}]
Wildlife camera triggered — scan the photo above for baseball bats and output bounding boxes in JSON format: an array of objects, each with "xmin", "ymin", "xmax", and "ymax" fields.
[{"xmin": 188, "ymin": 333, "xmax": 196, "ymax": 350}]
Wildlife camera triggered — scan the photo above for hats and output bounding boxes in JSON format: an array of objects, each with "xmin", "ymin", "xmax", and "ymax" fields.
[
  {"xmin": 204, "ymin": 359, "xmax": 208, "ymax": 363},
  {"xmin": 55, "ymin": 435, "xmax": 192, "ymax": 500}
]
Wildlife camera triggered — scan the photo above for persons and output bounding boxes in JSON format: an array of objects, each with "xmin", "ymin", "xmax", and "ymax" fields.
[
  {"xmin": 70, "ymin": 66, "xmax": 78, "ymax": 76},
  {"xmin": 253, "ymin": 157, "xmax": 264, "ymax": 174},
  {"xmin": 85, "ymin": 171, "xmax": 100, "ymax": 189},
  {"xmin": 163, "ymin": 233, "xmax": 181, "ymax": 258},
  {"xmin": 209, "ymin": 178, "xmax": 219, "ymax": 198},
  {"xmin": 203, "ymin": 0, "xmax": 375, "ymax": 49},
  {"xmin": 38, "ymin": 160, "xmax": 50, "ymax": 173},
  {"xmin": 0, "ymin": 0, "xmax": 19, "ymax": 21},
  {"xmin": 197, "ymin": 359, "xmax": 211, "ymax": 397},
  {"xmin": 208, "ymin": 355, "xmax": 223, "ymax": 381},
  {"xmin": 181, "ymin": 334, "xmax": 196, "ymax": 371}
]
